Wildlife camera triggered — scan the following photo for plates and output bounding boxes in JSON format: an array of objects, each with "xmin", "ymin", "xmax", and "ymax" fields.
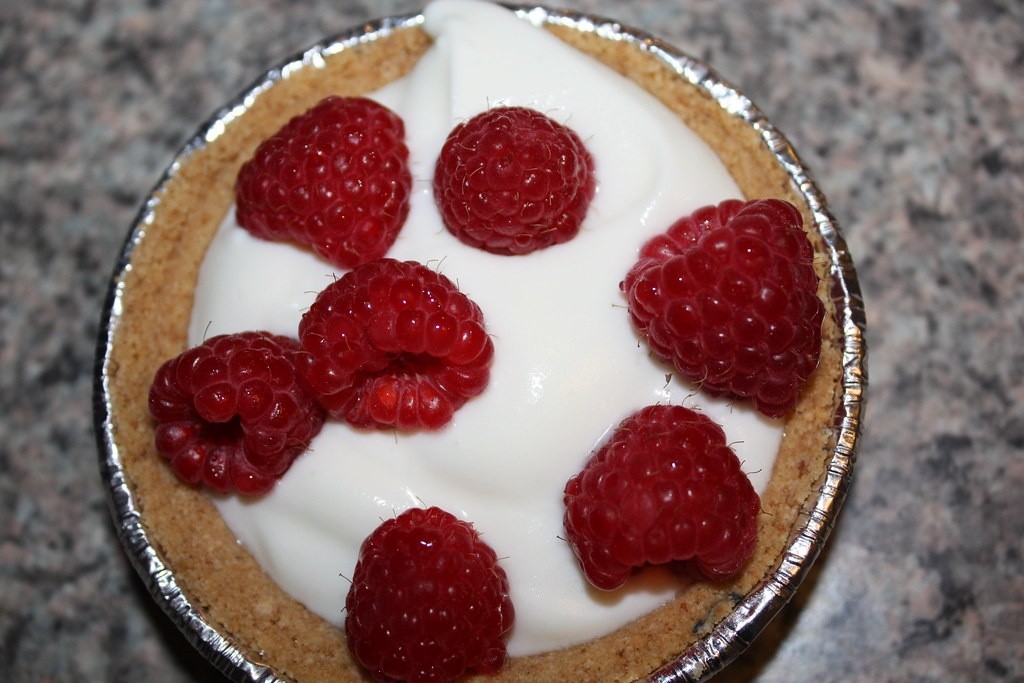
[{"xmin": 95, "ymin": 4, "xmax": 864, "ymax": 683}]
